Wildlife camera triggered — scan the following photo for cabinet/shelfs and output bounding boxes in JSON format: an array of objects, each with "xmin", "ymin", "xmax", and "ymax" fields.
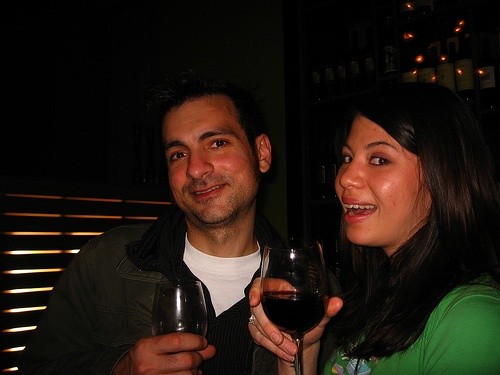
[{"xmin": 300, "ymin": 0, "xmax": 500, "ymax": 244}]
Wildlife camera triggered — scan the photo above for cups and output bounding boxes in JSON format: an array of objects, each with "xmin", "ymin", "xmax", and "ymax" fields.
[{"xmin": 151, "ymin": 278, "xmax": 208, "ymax": 338}]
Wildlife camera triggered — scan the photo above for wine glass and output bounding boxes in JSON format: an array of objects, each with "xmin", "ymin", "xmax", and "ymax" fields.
[{"xmin": 260, "ymin": 237, "xmax": 328, "ymax": 375}]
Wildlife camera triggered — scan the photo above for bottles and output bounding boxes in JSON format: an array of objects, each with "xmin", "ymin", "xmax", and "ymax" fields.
[
  {"xmin": 401, "ymin": 40, "xmax": 418, "ymax": 84},
  {"xmin": 477, "ymin": 38, "xmax": 500, "ymax": 109},
  {"xmin": 363, "ymin": 43, "xmax": 378, "ymax": 90},
  {"xmin": 417, "ymin": 32, "xmax": 436, "ymax": 83},
  {"xmin": 437, "ymin": 35, "xmax": 455, "ymax": 92},
  {"xmin": 349, "ymin": 49, "xmax": 363, "ymax": 93},
  {"xmin": 311, "ymin": 59, "xmax": 324, "ymax": 102},
  {"xmin": 455, "ymin": 36, "xmax": 477, "ymax": 91},
  {"xmin": 324, "ymin": 57, "xmax": 336, "ymax": 99},
  {"xmin": 335, "ymin": 56, "xmax": 349, "ymax": 95},
  {"xmin": 382, "ymin": 16, "xmax": 398, "ymax": 74}
]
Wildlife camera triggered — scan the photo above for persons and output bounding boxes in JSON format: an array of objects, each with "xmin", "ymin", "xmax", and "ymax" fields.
[
  {"xmin": 17, "ymin": 83, "xmax": 336, "ymax": 375},
  {"xmin": 247, "ymin": 80, "xmax": 500, "ymax": 375}
]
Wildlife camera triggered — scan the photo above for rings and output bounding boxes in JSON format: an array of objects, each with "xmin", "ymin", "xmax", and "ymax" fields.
[{"xmin": 246, "ymin": 314, "xmax": 257, "ymax": 325}]
